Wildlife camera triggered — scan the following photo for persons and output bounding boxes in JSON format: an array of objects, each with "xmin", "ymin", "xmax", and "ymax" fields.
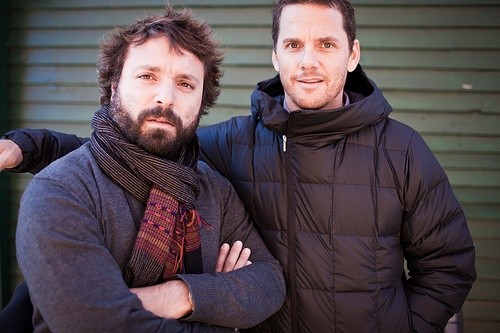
[
  {"xmin": 1, "ymin": 0, "xmax": 476, "ymax": 332},
  {"xmin": 14, "ymin": 0, "xmax": 286, "ymax": 332}
]
